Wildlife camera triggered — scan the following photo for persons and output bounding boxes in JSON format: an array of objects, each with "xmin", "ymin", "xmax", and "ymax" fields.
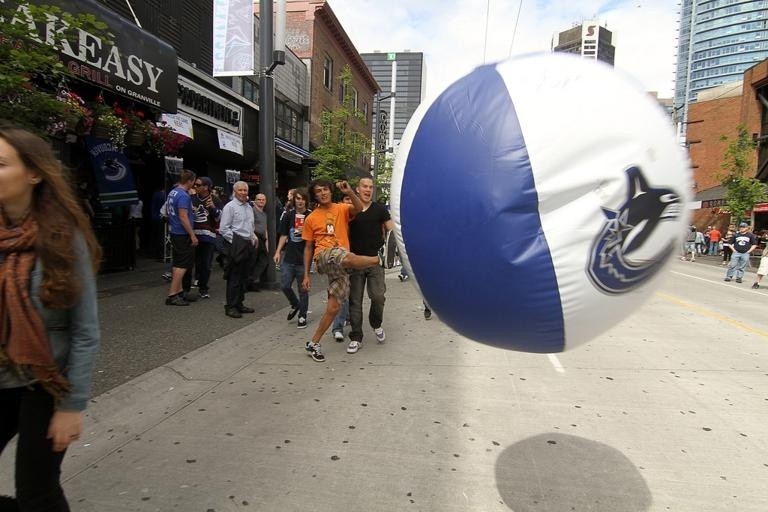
[
  {"xmin": 273, "ymin": 173, "xmax": 431, "ymax": 362},
  {"xmin": 0, "ymin": 126, "xmax": 102, "ymax": 511},
  {"xmin": 160, "ymin": 169, "xmax": 268, "ymax": 318},
  {"xmin": 679, "ymin": 222, "xmax": 768, "ymax": 289}
]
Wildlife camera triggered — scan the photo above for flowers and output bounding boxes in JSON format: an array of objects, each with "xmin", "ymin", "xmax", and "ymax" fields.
[{"xmin": 52, "ymin": 93, "xmax": 175, "ymax": 148}]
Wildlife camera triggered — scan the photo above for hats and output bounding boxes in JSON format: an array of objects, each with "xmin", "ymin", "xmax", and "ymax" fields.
[{"xmin": 740, "ymin": 223, "xmax": 747, "ymax": 227}]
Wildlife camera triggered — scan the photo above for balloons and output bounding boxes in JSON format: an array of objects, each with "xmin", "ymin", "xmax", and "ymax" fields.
[{"xmin": 390, "ymin": 52, "xmax": 694, "ymax": 357}]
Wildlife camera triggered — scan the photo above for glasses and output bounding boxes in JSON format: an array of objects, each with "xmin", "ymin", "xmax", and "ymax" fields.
[{"xmin": 194, "ymin": 183, "xmax": 202, "ymax": 187}]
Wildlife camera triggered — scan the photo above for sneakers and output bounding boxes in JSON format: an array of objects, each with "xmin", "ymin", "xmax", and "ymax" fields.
[
  {"xmin": 347, "ymin": 341, "xmax": 362, "ymax": 353},
  {"xmin": 736, "ymin": 277, "xmax": 742, "ymax": 283},
  {"xmin": 238, "ymin": 305, "xmax": 254, "ymax": 313},
  {"xmin": 163, "ymin": 272, "xmax": 172, "ymax": 281},
  {"xmin": 752, "ymin": 282, "xmax": 759, "ymax": 289},
  {"xmin": 377, "ymin": 230, "xmax": 396, "ymax": 269},
  {"xmin": 225, "ymin": 307, "xmax": 241, "ymax": 318},
  {"xmin": 199, "ymin": 289, "xmax": 209, "ymax": 298},
  {"xmin": 424, "ymin": 306, "xmax": 431, "ymax": 319},
  {"xmin": 725, "ymin": 277, "xmax": 732, "ymax": 281},
  {"xmin": 398, "ymin": 274, "xmax": 404, "ymax": 281},
  {"xmin": 374, "ymin": 327, "xmax": 385, "ymax": 342},
  {"xmin": 166, "ymin": 290, "xmax": 197, "ymax": 305},
  {"xmin": 303, "ymin": 342, "xmax": 325, "ymax": 361},
  {"xmin": 287, "ymin": 304, "xmax": 300, "ymax": 320},
  {"xmin": 334, "ymin": 332, "xmax": 343, "ymax": 340},
  {"xmin": 297, "ymin": 314, "xmax": 307, "ymax": 328}
]
